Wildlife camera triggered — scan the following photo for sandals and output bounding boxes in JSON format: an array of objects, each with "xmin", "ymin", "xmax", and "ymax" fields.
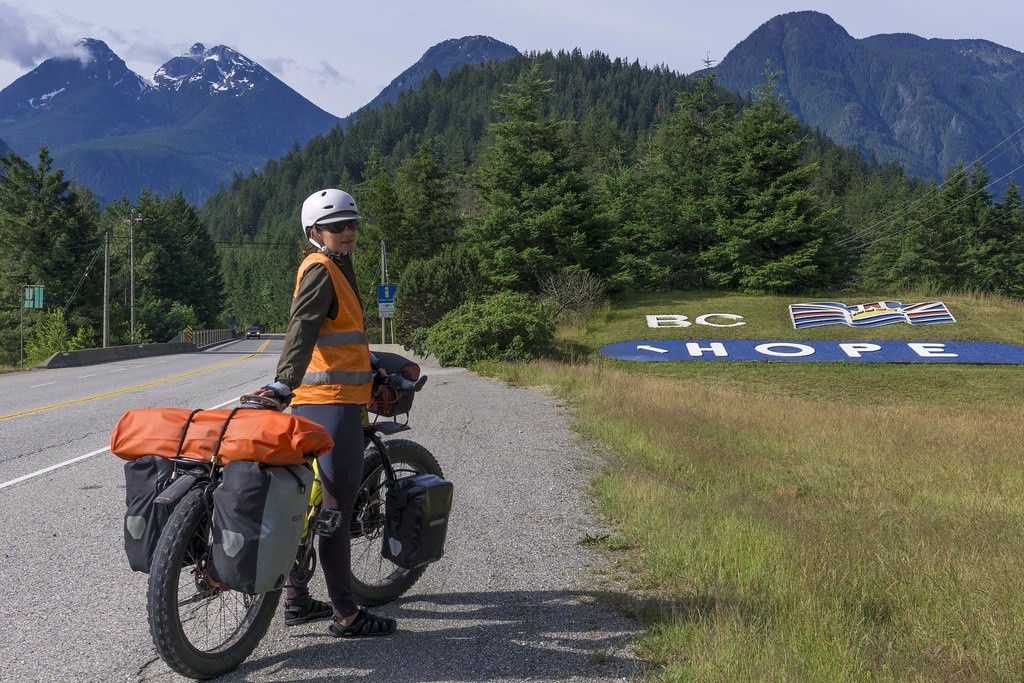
[
  {"xmin": 283, "ymin": 597, "xmax": 335, "ymax": 625},
  {"xmin": 328, "ymin": 608, "xmax": 397, "ymax": 637}
]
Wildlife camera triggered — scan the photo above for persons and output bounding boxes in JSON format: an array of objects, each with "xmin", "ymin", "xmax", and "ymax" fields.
[{"xmin": 247, "ymin": 188, "xmax": 398, "ymax": 639}]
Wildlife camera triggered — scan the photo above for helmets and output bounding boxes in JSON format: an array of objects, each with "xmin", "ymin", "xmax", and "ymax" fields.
[{"xmin": 301, "ymin": 189, "xmax": 362, "ymax": 240}]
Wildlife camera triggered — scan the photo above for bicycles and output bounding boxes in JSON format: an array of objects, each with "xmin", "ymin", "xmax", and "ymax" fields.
[{"xmin": 143, "ymin": 372, "xmax": 447, "ymax": 682}]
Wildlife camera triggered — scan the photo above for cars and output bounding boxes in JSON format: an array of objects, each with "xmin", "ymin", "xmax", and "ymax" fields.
[{"xmin": 246, "ymin": 327, "xmax": 261, "ymax": 340}]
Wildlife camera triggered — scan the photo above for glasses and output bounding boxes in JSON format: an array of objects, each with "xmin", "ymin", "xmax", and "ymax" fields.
[{"xmin": 319, "ymin": 219, "xmax": 359, "ymax": 233}]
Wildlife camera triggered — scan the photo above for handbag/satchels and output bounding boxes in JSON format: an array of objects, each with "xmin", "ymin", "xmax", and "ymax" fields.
[
  {"xmin": 110, "ymin": 405, "xmax": 336, "ymax": 597},
  {"xmin": 381, "ymin": 472, "xmax": 454, "ymax": 569}
]
